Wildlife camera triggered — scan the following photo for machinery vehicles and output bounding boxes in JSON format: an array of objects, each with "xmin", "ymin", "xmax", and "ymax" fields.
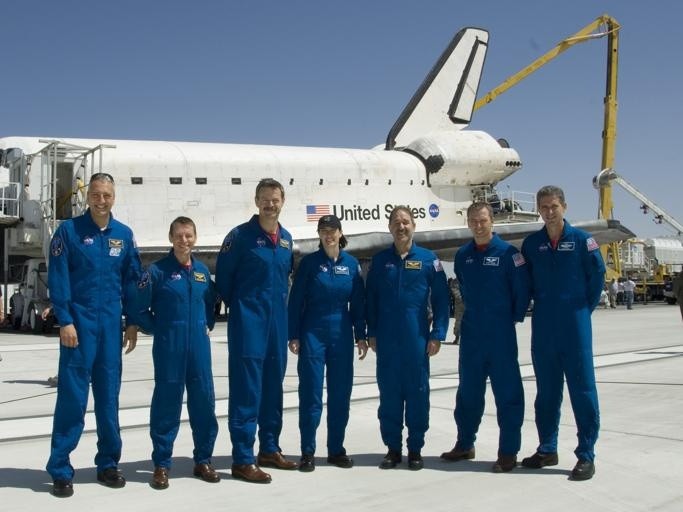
[
  {"xmin": 472, "ymin": 14, "xmax": 670, "ymax": 302},
  {"xmin": 591, "ymin": 164, "xmax": 682, "ymax": 306}
]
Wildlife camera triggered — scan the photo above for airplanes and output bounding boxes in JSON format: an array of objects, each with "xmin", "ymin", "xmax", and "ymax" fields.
[{"xmin": 0, "ymin": 26, "xmax": 636, "ymax": 331}]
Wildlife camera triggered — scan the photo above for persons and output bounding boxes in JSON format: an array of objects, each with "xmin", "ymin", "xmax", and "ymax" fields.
[
  {"xmin": 451, "ymin": 274, "xmax": 466, "ymax": 345},
  {"xmin": 47, "ymin": 173, "xmax": 140, "ymax": 498},
  {"xmin": 216, "ymin": 179, "xmax": 298, "ymax": 485},
  {"xmin": 0, "ymin": 286, "xmax": 4, "ymax": 361},
  {"xmin": 288, "ymin": 215, "xmax": 366, "ymax": 472},
  {"xmin": 137, "ymin": 217, "xmax": 220, "ymax": 490},
  {"xmin": 442, "ymin": 203, "xmax": 531, "ymax": 474},
  {"xmin": 521, "ymin": 187, "xmax": 606, "ymax": 480},
  {"xmin": 41, "ymin": 300, "xmax": 54, "ymax": 321},
  {"xmin": 365, "ymin": 208, "xmax": 450, "ymax": 470},
  {"xmin": 609, "ymin": 274, "xmax": 636, "ymax": 310}
]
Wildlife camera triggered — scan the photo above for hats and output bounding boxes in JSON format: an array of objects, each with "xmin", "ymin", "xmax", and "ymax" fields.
[{"xmin": 317, "ymin": 215, "xmax": 341, "ymax": 229}]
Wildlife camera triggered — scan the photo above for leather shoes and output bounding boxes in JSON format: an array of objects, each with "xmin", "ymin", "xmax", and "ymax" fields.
[
  {"xmin": 441, "ymin": 448, "xmax": 476, "ymax": 463},
  {"xmin": 257, "ymin": 449, "xmax": 298, "ymax": 469},
  {"xmin": 379, "ymin": 451, "xmax": 402, "ymax": 469},
  {"xmin": 193, "ymin": 463, "xmax": 219, "ymax": 483},
  {"xmin": 327, "ymin": 454, "xmax": 354, "ymax": 468},
  {"xmin": 521, "ymin": 452, "xmax": 558, "ymax": 468},
  {"xmin": 151, "ymin": 467, "xmax": 170, "ymax": 490},
  {"xmin": 494, "ymin": 454, "xmax": 517, "ymax": 472},
  {"xmin": 571, "ymin": 459, "xmax": 596, "ymax": 480},
  {"xmin": 97, "ymin": 469, "xmax": 125, "ymax": 488},
  {"xmin": 408, "ymin": 451, "xmax": 424, "ymax": 471},
  {"xmin": 53, "ymin": 479, "xmax": 73, "ymax": 497},
  {"xmin": 299, "ymin": 451, "xmax": 316, "ymax": 471},
  {"xmin": 232, "ymin": 462, "xmax": 272, "ymax": 483}
]
